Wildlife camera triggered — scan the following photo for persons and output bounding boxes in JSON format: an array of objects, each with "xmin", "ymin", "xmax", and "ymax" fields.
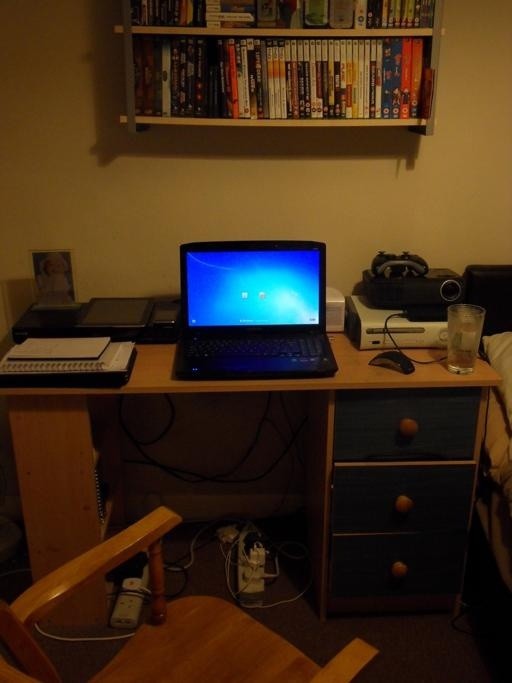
[{"xmin": 34, "ymin": 252, "xmax": 74, "ymax": 307}]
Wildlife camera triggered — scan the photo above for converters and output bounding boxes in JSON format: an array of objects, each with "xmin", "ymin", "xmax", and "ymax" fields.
[
  {"xmin": 218, "ymin": 525, "xmax": 240, "ymax": 543},
  {"xmin": 249, "ymin": 548, "xmax": 267, "ymax": 568},
  {"xmin": 106, "ymin": 552, "xmax": 146, "ymax": 581},
  {"xmin": 243, "ymin": 532, "xmax": 260, "ymax": 556}
]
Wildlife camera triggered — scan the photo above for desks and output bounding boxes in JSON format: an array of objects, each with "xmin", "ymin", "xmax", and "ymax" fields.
[{"xmin": 0, "ymin": 332, "xmax": 502, "ymax": 628}]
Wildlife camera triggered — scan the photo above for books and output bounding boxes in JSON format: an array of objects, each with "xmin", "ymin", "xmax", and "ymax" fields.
[
  {"xmin": 132, "ymin": 33, "xmax": 436, "ymax": 119},
  {"xmin": 7, "ymin": 337, "xmax": 112, "ymax": 361},
  {"xmin": 1, "ymin": 341, "xmax": 121, "ymax": 372},
  {"xmin": 133, "ymin": 0, "xmax": 437, "ymax": 29},
  {"xmin": 0, "ymin": 340, "xmax": 136, "ymax": 376}
]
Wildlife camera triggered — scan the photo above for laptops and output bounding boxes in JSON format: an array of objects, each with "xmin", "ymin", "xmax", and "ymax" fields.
[{"xmin": 173, "ymin": 238, "xmax": 340, "ymax": 380}]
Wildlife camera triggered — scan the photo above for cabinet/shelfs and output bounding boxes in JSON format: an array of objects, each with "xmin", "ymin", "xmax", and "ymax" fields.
[
  {"xmin": 308, "ymin": 390, "xmax": 487, "ymax": 628},
  {"xmin": 113, "ymin": 0, "xmax": 446, "ymax": 137}
]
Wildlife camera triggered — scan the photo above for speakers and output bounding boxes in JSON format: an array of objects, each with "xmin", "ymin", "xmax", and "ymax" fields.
[{"xmin": 361, "ymin": 266, "xmax": 469, "ymax": 322}]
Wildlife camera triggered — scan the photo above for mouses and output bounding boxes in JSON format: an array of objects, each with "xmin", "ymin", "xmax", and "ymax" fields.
[{"xmin": 368, "ymin": 350, "xmax": 416, "ymax": 375}]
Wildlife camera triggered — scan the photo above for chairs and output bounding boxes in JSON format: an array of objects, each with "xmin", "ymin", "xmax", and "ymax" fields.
[{"xmin": 0, "ymin": 495, "xmax": 383, "ymax": 682}]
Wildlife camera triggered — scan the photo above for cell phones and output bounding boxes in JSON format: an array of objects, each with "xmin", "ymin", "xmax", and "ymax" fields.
[{"xmin": 153, "ymin": 301, "xmax": 178, "ymax": 324}]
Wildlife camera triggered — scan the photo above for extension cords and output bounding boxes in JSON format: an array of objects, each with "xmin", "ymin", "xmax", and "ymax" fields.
[
  {"xmin": 237, "ymin": 529, "xmax": 266, "ymax": 603},
  {"xmin": 110, "ymin": 546, "xmax": 150, "ymax": 630}
]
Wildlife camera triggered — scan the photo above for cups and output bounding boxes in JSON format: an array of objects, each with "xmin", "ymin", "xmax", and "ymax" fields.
[{"xmin": 444, "ymin": 303, "xmax": 487, "ymax": 375}]
